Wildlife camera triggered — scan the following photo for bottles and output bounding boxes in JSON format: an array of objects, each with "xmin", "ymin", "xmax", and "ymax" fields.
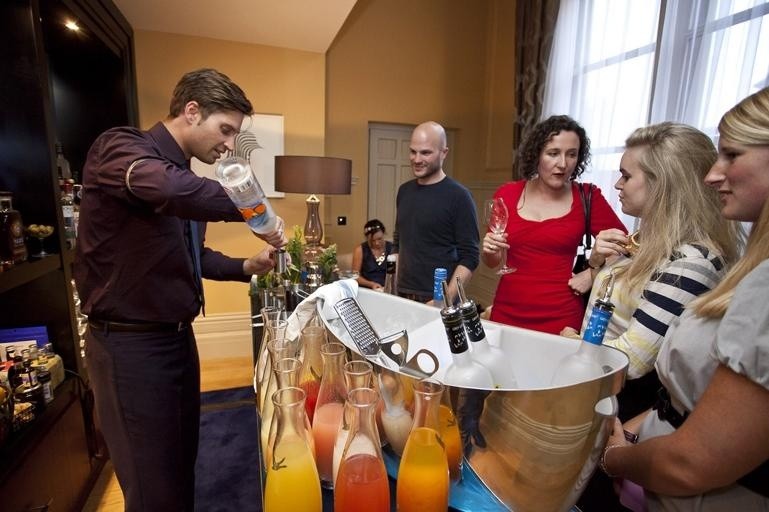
[
  {"xmin": 461, "ymin": 299, "xmax": 521, "ymax": 391},
  {"xmin": 0, "ymin": 343, "xmax": 67, "ymax": 415},
  {"xmin": 381, "ymin": 255, "xmax": 399, "ymax": 297},
  {"xmin": 58, "ymin": 171, "xmax": 83, "ymax": 250},
  {"xmin": 0, "ymin": 190, "xmax": 29, "ymax": 265},
  {"xmin": 581, "ymin": 271, "xmax": 616, "ymax": 346},
  {"xmin": 214, "ymin": 156, "xmax": 277, "ymax": 236},
  {"xmin": 432, "ymin": 267, "xmax": 448, "ymax": 308},
  {"xmin": 252, "ymin": 282, "xmax": 464, "ymax": 512},
  {"xmin": 437, "ymin": 309, "xmax": 494, "ymax": 390}
]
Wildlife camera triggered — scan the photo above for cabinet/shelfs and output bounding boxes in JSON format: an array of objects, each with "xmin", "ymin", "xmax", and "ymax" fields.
[
  {"xmin": 30, "ymin": 1, "xmax": 142, "ymax": 495},
  {"xmin": 3, "ymin": 0, "xmax": 96, "ymax": 510}
]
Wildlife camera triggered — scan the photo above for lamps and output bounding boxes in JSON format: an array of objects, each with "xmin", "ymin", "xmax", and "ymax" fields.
[{"xmin": 273, "ymin": 151, "xmax": 352, "ymax": 263}]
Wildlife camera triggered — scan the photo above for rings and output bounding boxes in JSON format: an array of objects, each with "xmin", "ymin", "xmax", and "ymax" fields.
[
  {"xmin": 489, "ymin": 245, "xmax": 493, "ymax": 250},
  {"xmin": 279, "ymin": 229, "xmax": 284, "ymax": 233}
]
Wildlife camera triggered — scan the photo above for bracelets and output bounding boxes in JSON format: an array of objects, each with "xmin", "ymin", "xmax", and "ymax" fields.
[
  {"xmin": 587, "ymin": 258, "xmax": 606, "ymax": 269},
  {"xmin": 611, "ymin": 426, "xmax": 639, "ymax": 445},
  {"xmin": 598, "ymin": 443, "xmax": 624, "ymax": 479}
]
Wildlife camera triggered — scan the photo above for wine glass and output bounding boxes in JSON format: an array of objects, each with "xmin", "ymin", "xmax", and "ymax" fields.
[
  {"xmin": 27, "ymin": 225, "xmax": 55, "ymax": 257},
  {"xmin": 484, "ymin": 197, "xmax": 517, "ymax": 277}
]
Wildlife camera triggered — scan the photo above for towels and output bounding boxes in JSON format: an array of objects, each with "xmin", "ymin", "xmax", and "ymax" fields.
[{"xmin": 283, "ymin": 277, "xmax": 362, "ymax": 344}]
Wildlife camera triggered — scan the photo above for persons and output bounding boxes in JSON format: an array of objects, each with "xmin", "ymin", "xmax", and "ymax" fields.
[
  {"xmin": 352, "ymin": 219, "xmax": 394, "ymax": 291},
  {"xmin": 599, "ymin": 83, "xmax": 769, "ymax": 512},
  {"xmin": 561, "ymin": 120, "xmax": 748, "ymax": 512},
  {"xmin": 388, "ymin": 121, "xmax": 481, "ymax": 310},
  {"xmin": 481, "ymin": 114, "xmax": 629, "ymax": 340},
  {"xmin": 74, "ymin": 65, "xmax": 292, "ymax": 511}
]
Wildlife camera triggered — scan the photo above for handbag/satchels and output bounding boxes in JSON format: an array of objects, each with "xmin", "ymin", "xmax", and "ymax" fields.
[{"xmin": 571, "ymin": 183, "xmax": 600, "ymax": 311}]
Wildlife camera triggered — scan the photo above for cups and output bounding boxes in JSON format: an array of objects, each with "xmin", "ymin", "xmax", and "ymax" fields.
[
  {"xmin": 467, "ymin": 295, "xmax": 481, "ymax": 318},
  {"xmin": 273, "ymin": 249, "xmax": 288, "ymax": 273}
]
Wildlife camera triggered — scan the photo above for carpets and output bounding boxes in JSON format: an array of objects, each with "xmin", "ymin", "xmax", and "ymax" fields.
[{"xmin": 189, "ymin": 383, "xmax": 264, "ymax": 512}]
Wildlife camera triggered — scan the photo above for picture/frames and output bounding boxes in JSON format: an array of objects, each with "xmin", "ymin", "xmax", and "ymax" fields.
[{"xmin": 191, "ymin": 111, "xmax": 288, "ymax": 198}]
